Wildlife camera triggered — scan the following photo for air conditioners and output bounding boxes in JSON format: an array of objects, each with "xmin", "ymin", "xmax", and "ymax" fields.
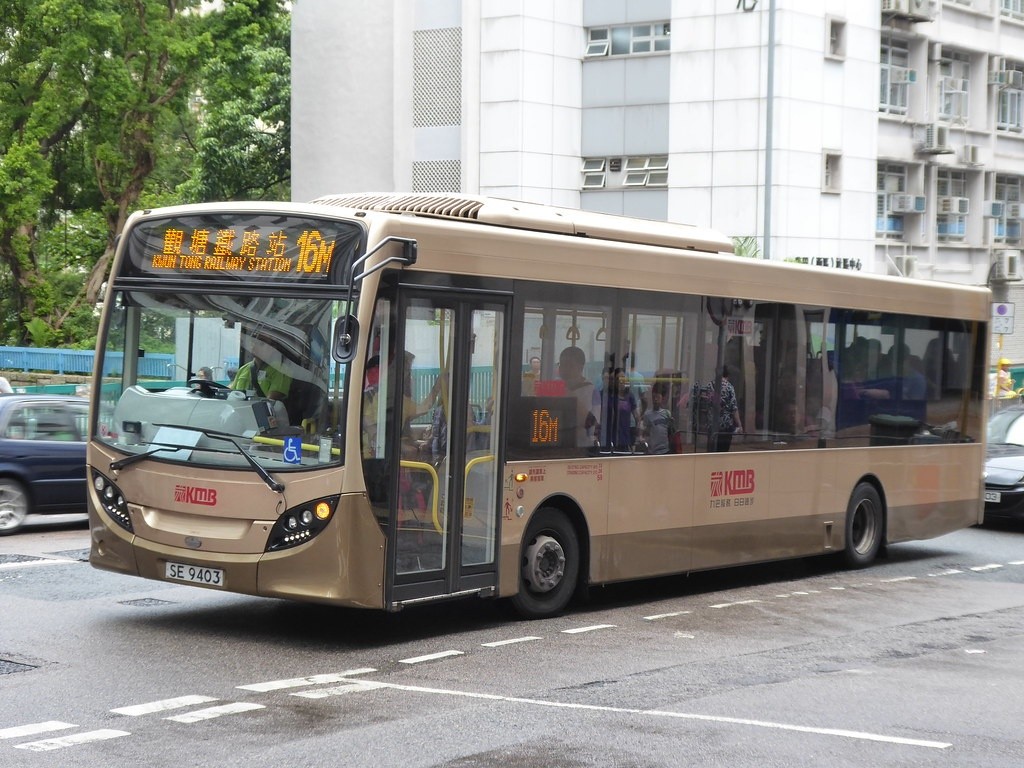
[
  {"xmin": 881, "ymin": 0, "xmax": 935, "ymax": 22},
  {"xmin": 924, "ymin": 121, "xmax": 950, "ymax": 153},
  {"xmin": 962, "ymin": 144, "xmax": 985, "ymax": 166},
  {"xmin": 1005, "ymin": 69, "xmax": 1023, "ymax": 87},
  {"xmin": 995, "ymin": 249, "xmax": 1022, "ymax": 281},
  {"xmin": 894, "ymin": 254, "xmax": 919, "ymax": 278}
]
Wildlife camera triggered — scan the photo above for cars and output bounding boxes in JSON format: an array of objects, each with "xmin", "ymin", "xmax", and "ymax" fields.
[
  {"xmin": 972, "ymin": 403, "xmax": 1024, "ymax": 535},
  {"xmin": 0, "ymin": 394, "xmax": 120, "ymax": 536}
]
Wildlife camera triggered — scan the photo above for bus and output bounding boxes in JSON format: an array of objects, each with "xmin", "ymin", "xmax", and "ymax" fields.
[{"xmin": 86, "ymin": 193, "xmax": 992, "ymax": 621}]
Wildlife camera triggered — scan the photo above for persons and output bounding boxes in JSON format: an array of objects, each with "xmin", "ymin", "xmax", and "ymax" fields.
[
  {"xmin": 997, "ymin": 359, "xmax": 1016, "ymax": 399},
  {"xmin": 229, "ymin": 327, "xmax": 293, "ymax": 410},
  {"xmin": 226, "ymin": 367, "xmax": 240, "ymax": 393},
  {"xmin": 356, "ymin": 302, "xmax": 978, "ymax": 551},
  {"xmin": 194, "ymin": 367, "xmax": 214, "ymax": 388}
]
